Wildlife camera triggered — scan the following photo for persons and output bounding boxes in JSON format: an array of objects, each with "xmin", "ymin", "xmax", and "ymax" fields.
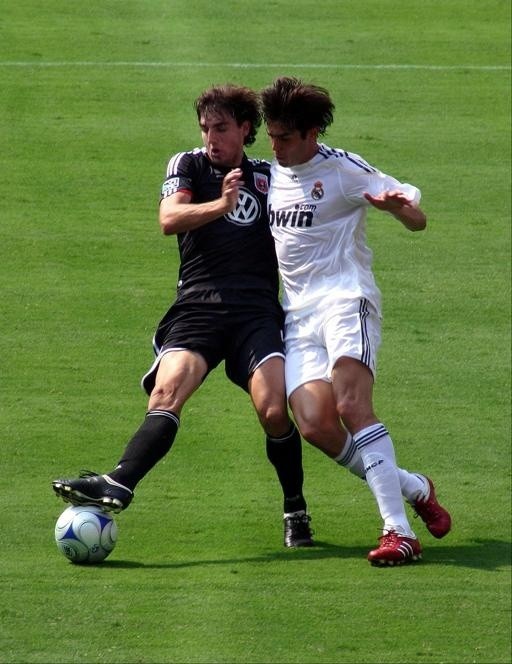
[
  {"xmin": 254, "ymin": 74, "xmax": 452, "ymax": 565},
  {"xmin": 51, "ymin": 80, "xmax": 314, "ymax": 551}
]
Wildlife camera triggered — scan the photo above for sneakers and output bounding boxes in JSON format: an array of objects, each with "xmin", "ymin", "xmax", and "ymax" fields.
[
  {"xmin": 406, "ymin": 473, "xmax": 452, "ymax": 538},
  {"xmin": 52, "ymin": 469, "xmax": 134, "ymax": 514},
  {"xmin": 368, "ymin": 528, "xmax": 423, "ymax": 564},
  {"xmin": 284, "ymin": 514, "xmax": 314, "ymax": 547}
]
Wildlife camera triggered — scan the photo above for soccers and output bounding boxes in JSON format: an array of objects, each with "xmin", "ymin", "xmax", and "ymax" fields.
[{"xmin": 55, "ymin": 504, "xmax": 118, "ymax": 565}]
